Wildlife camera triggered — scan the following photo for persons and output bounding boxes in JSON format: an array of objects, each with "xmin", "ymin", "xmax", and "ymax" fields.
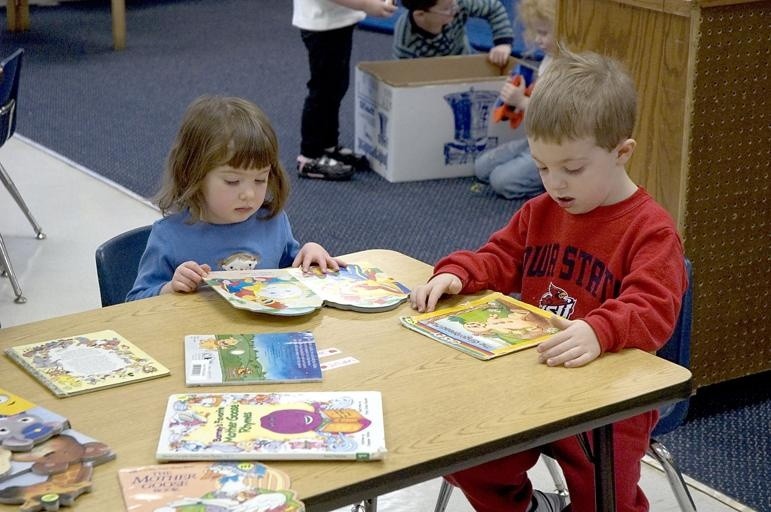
[
  {"xmin": 392, "ymin": 1, "xmax": 514, "ymax": 67},
  {"xmin": 519, "ymin": 0, "xmax": 559, "ymax": 51},
  {"xmin": 291, "ymin": 0, "xmax": 398, "ymax": 181},
  {"xmin": 124, "ymin": 94, "xmax": 348, "ymax": 303},
  {"xmin": 408, "ymin": 46, "xmax": 689, "ymax": 511},
  {"xmin": 474, "ymin": 81, "xmax": 547, "ymax": 201}
]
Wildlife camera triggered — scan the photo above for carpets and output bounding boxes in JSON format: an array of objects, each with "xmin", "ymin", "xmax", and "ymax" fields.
[{"xmin": 2, "ymin": 0, "xmax": 770, "ymax": 512}]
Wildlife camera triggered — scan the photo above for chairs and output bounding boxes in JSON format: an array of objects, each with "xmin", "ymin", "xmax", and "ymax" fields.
[
  {"xmin": 94, "ymin": 222, "xmax": 380, "ymax": 512},
  {"xmin": 1, "ymin": 46, "xmax": 46, "ymax": 306},
  {"xmin": 429, "ymin": 251, "xmax": 700, "ymax": 511}
]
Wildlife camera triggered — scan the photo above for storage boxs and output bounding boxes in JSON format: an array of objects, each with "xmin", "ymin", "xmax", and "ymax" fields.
[{"xmin": 349, "ymin": 47, "xmax": 538, "ymax": 188}]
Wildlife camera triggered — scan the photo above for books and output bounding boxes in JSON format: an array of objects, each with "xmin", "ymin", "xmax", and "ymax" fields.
[
  {"xmin": 3, "ymin": 329, "xmax": 172, "ymax": 399},
  {"xmin": 201, "ymin": 260, "xmax": 411, "ymax": 317},
  {"xmin": 155, "ymin": 388, "xmax": 387, "ymax": 463},
  {"xmin": 400, "ymin": 292, "xmax": 573, "ymax": 360},
  {"xmin": 184, "ymin": 331, "xmax": 323, "ymax": 385}
]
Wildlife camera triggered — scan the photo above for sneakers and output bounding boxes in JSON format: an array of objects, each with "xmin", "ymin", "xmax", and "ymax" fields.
[
  {"xmin": 533, "ymin": 489, "xmax": 566, "ymax": 511},
  {"xmin": 297, "ymin": 155, "xmax": 355, "ymax": 181},
  {"xmin": 301, "ymin": 146, "xmax": 369, "ymax": 171}
]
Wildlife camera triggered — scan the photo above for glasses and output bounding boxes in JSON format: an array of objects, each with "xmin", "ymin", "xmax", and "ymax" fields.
[{"xmin": 416, "ymin": 6, "xmax": 459, "ymax": 17}]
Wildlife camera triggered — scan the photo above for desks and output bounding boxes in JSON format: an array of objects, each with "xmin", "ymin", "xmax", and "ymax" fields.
[{"xmin": 1, "ymin": 246, "xmax": 695, "ymax": 509}]
